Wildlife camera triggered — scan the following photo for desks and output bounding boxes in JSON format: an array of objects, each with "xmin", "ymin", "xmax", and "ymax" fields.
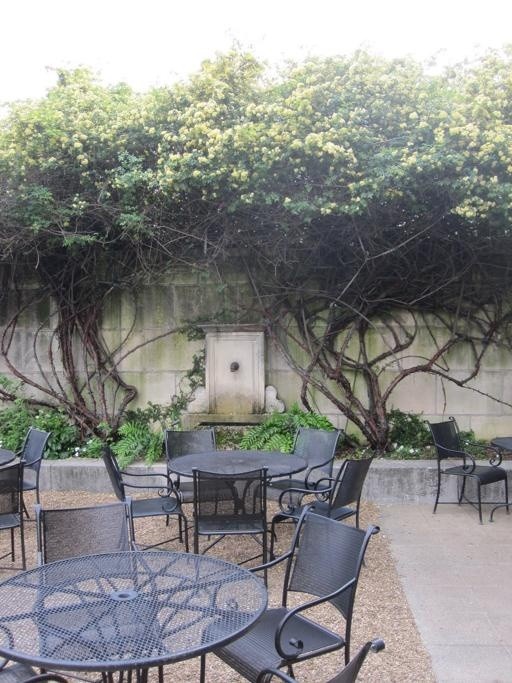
[
  {"xmin": 1, "ymin": 448, "xmax": 14, "ymax": 468},
  {"xmin": 489, "ymin": 437, "xmax": 510, "ymax": 522},
  {"xmin": 169, "ymin": 450, "xmax": 309, "ymax": 542},
  {"xmin": 2, "ymin": 551, "xmax": 271, "ymax": 683}
]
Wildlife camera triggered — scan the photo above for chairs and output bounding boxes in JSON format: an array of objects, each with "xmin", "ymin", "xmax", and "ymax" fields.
[
  {"xmin": 331, "ymin": 638, "xmax": 385, "ymax": 682},
  {"xmin": 162, "ymin": 427, "xmax": 221, "ymax": 525},
  {"xmin": 200, "ymin": 504, "xmax": 381, "ymax": 681},
  {"xmin": 1, "ymin": 627, "xmax": 66, "ymax": 682},
  {"xmin": 8, "ymin": 428, "xmax": 50, "ymax": 523},
  {"xmin": 270, "ymin": 452, "xmax": 378, "ymax": 562},
  {"xmin": 423, "ymin": 418, "xmax": 510, "ymax": 524},
  {"xmin": 36, "ymin": 500, "xmax": 163, "ymax": 680},
  {"xmin": 100, "ymin": 447, "xmax": 190, "ymax": 554},
  {"xmin": 192, "ymin": 466, "xmax": 269, "ymax": 589},
  {"xmin": 252, "ymin": 427, "xmax": 344, "ymax": 528},
  {"xmin": 1, "ymin": 460, "xmax": 27, "ymax": 571}
]
